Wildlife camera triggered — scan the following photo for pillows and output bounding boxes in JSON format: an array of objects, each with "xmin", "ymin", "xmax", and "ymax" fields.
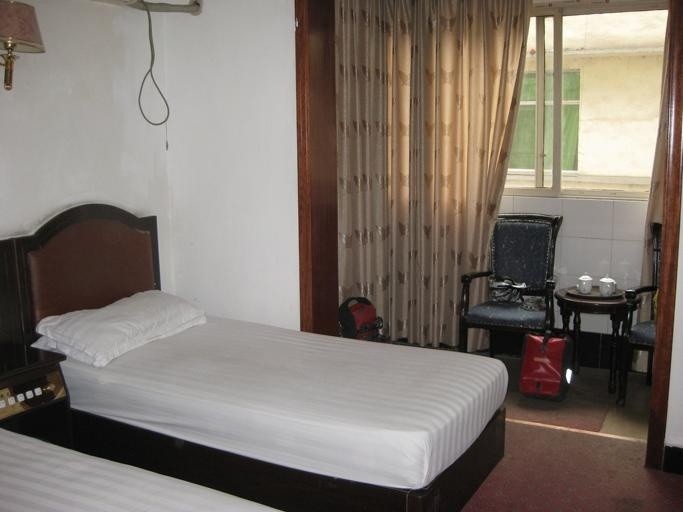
[
  {"xmin": 29, "ymin": 317, "xmax": 206, "ymax": 367},
  {"xmin": 34, "ymin": 290, "xmax": 206, "ymax": 367}
]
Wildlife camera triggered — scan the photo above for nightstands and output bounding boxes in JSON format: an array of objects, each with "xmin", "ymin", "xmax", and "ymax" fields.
[{"xmin": 0, "ymin": 343, "xmax": 71, "ymax": 445}]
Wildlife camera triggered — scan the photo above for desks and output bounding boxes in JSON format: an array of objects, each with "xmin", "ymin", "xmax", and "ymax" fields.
[{"xmin": 555, "ymin": 287, "xmax": 643, "ymax": 393}]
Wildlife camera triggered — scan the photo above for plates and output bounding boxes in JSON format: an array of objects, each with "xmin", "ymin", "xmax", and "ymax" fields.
[{"xmin": 567, "ymin": 286, "xmax": 626, "ymax": 299}]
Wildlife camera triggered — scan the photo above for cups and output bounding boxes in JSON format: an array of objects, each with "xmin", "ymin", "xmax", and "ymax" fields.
[
  {"xmin": 576, "ymin": 271, "xmax": 594, "ymax": 294},
  {"xmin": 598, "ymin": 274, "xmax": 616, "ymax": 296}
]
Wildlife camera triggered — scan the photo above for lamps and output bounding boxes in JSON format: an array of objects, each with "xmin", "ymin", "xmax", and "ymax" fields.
[{"xmin": 1, "ymin": 0, "xmax": 45, "ymax": 90}]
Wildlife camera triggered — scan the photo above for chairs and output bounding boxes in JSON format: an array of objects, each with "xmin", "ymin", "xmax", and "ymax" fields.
[
  {"xmin": 460, "ymin": 214, "xmax": 563, "ymax": 358},
  {"xmin": 616, "ymin": 221, "xmax": 662, "ymax": 408}
]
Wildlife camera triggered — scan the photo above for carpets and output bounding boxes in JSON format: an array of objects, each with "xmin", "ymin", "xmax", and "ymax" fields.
[
  {"xmin": 461, "ymin": 418, "xmax": 683, "ymax": 510},
  {"xmin": 476, "ymin": 351, "xmax": 645, "ymax": 432}
]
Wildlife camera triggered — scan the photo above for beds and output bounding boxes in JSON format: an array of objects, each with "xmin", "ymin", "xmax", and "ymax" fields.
[
  {"xmin": 12, "ymin": 204, "xmax": 510, "ymax": 512},
  {"xmin": 0, "ymin": 428, "xmax": 286, "ymax": 512}
]
